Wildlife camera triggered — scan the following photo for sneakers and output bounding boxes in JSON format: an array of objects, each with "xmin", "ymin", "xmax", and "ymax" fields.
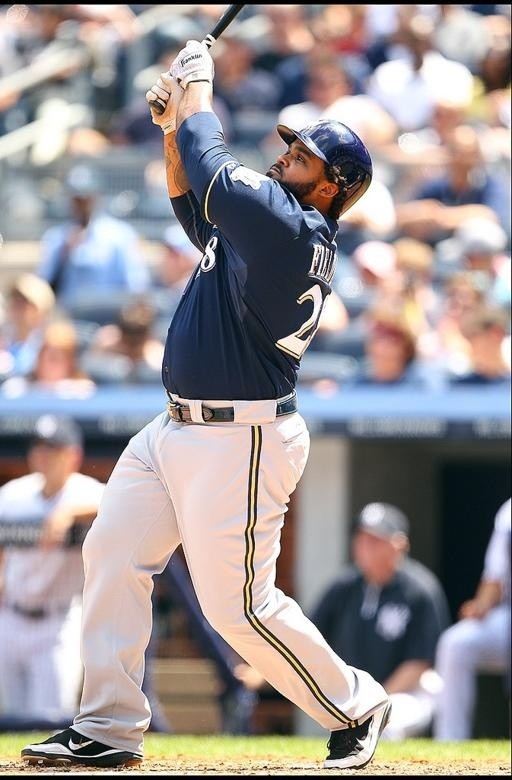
[
  {"xmin": 19, "ymin": 728, "xmax": 143, "ymax": 770},
  {"xmin": 324, "ymin": 701, "xmax": 392, "ymax": 769}
]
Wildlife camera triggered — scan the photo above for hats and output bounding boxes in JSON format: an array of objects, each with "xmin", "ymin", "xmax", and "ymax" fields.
[
  {"xmin": 351, "ymin": 502, "xmax": 412, "ymax": 545},
  {"xmin": 30, "ymin": 415, "xmax": 82, "ymax": 449}
]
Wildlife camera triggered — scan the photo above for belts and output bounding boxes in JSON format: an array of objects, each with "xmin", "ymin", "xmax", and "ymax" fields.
[{"xmin": 166, "ymin": 392, "xmax": 298, "ymax": 423}]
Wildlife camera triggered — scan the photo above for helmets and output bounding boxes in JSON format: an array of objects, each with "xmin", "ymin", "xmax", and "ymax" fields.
[{"xmin": 275, "ymin": 119, "xmax": 374, "ymax": 221}]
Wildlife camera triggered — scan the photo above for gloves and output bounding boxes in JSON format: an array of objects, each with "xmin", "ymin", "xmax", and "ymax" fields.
[
  {"xmin": 170, "ymin": 41, "xmax": 213, "ymax": 86},
  {"xmin": 146, "ymin": 70, "xmax": 183, "ymax": 136}
]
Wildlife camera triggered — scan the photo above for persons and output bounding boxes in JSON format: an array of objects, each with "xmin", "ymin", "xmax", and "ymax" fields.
[
  {"xmin": 2, "ymin": 415, "xmax": 105, "ymax": 730},
  {"xmin": 20, "ymin": 40, "xmax": 393, "ymax": 768},
  {"xmin": 0, "ymin": 2, "xmax": 510, "ymax": 396},
  {"xmin": 433, "ymin": 498, "xmax": 511, "ymax": 744},
  {"xmin": 231, "ymin": 503, "xmax": 450, "ymax": 744}
]
